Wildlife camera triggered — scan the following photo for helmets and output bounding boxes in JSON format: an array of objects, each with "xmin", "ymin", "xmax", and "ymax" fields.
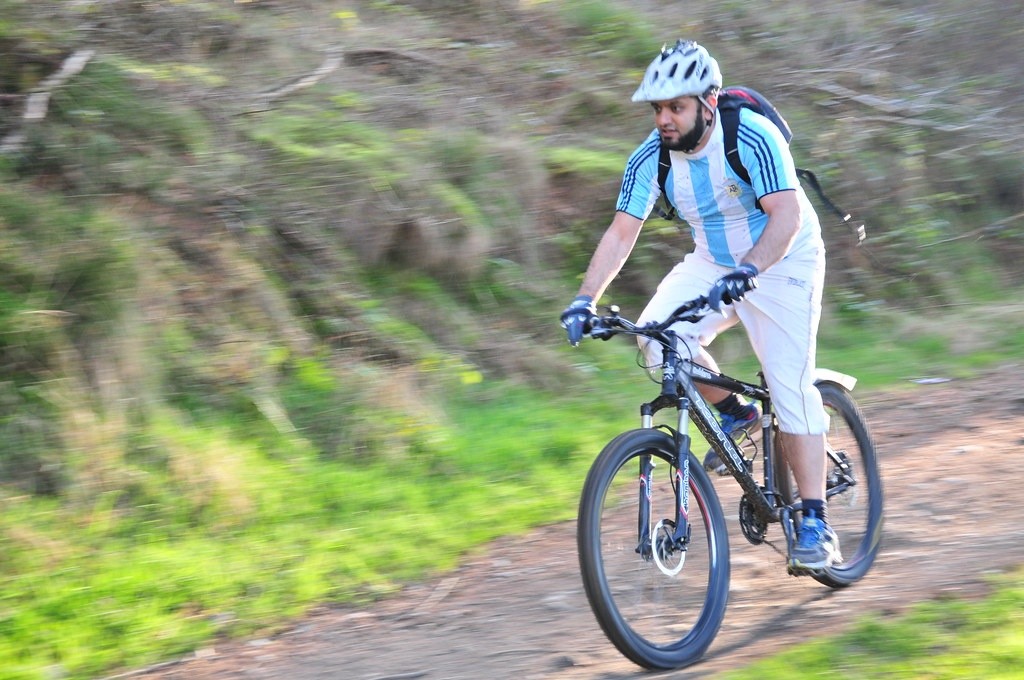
[{"xmin": 632, "ymin": 43, "xmax": 723, "ymax": 101}]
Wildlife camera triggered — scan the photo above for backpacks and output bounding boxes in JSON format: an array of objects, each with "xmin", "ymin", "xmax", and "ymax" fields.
[{"xmin": 656, "ymin": 85, "xmax": 793, "ymax": 203}]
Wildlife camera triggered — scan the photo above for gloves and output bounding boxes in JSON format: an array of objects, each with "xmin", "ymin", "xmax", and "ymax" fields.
[
  {"xmin": 560, "ymin": 295, "xmax": 597, "ymax": 347},
  {"xmin": 708, "ymin": 264, "xmax": 759, "ymax": 316}
]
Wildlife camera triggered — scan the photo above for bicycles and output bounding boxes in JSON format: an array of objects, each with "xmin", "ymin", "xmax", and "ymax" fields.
[{"xmin": 564, "ymin": 272, "xmax": 892, "ymax": 674}]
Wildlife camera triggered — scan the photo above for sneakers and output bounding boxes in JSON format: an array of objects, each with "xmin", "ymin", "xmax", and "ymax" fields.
[
  {"xmin": 704, "ymin": 403, "xmax": 764, "ymax": 473},
  {"xmin": 789, "ymin": 517, "xmax": 844, "ymax": 577}
]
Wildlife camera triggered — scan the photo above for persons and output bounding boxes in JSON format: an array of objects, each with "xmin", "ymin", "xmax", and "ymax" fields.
[{"xmin": 558, "ymin": 38, "xmax": 844, "ymax": 569}]
